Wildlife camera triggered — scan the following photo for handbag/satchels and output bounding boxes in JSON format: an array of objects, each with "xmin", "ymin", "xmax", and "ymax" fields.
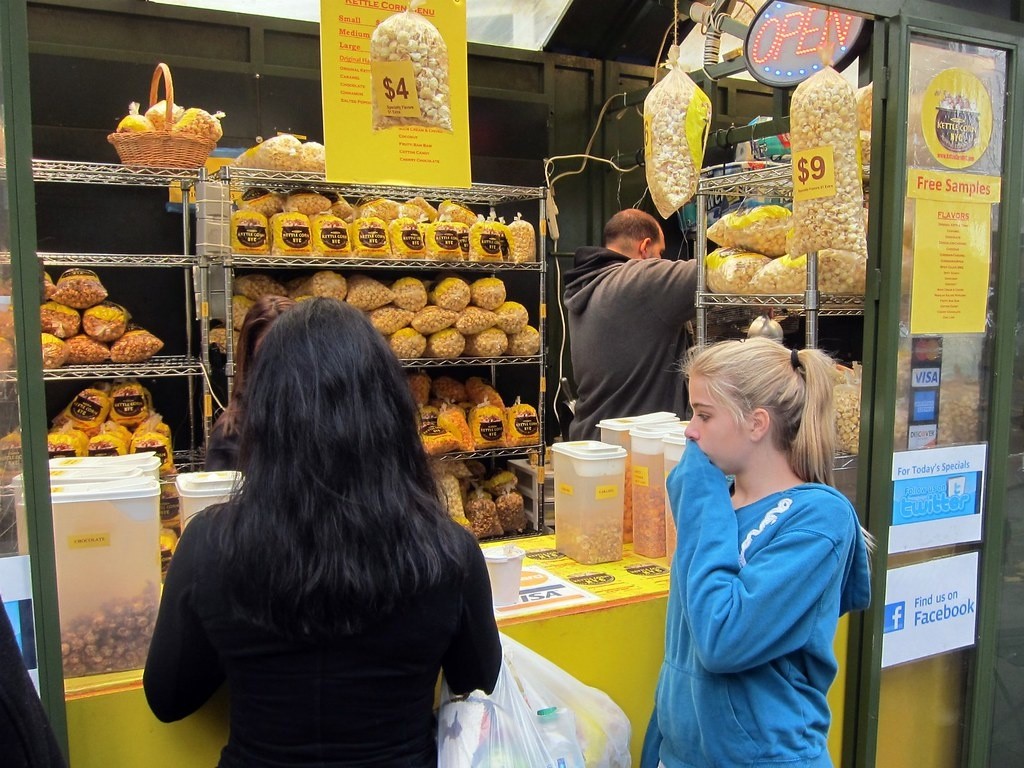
[{"xmin": 434, "ymin": 633, "xmax": 632, "ymax": 768}]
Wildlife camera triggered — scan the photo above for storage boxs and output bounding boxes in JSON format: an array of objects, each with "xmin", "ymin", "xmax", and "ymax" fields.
[
  {"xmin": 549, "ymin": 412, "xmax": 696, "ymax": 569},
  {"xmin": 14, "ymin": 450, "xmax": 248, "ymax": 678}
]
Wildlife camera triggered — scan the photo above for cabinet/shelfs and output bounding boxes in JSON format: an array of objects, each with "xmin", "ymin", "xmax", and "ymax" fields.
[
  {"xmin": 695, "ymin": 163, "xmax": 869, "ymax": 473},
  {"xmin": 0, "ymin": 156, "xmax": 207, "ymax": 475},
  {"xmin": 207, "ymin": 165, "xmax": 550, "ymax": 537}
]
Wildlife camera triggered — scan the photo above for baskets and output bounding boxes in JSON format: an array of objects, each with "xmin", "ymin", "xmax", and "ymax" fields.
[{"xmin": 107, "ymin": 63, "xmax": 216, "ymax": 171}]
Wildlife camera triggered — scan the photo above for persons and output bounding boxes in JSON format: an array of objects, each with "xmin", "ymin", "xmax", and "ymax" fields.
[
  {"xmin": 638, "ymin": 337, "xmax": 874, "ymax": 768},
  {"xmin": 141, "ymin": 295, "xmax": 504, "ymax": 768},
  {"xmin": 565, "ymin": 208, "xmax": 709, "ymax": 440},
  {"xmin": 205, "ymin": 295, "xmax": 298, "ymax": 469}
]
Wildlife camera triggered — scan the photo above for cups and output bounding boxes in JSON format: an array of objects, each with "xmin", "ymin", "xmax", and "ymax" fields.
[{"xmin": 483, "ymin": 546, "xmax": 525, "ymax": 605}]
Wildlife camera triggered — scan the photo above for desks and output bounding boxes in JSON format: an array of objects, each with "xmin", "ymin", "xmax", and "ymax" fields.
[{"xmin": 47, "ymin": 533, "xmax": 970, "ymax": 768}]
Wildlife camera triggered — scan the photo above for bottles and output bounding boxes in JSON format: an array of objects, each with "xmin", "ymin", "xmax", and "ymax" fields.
[{"xmin": 537, "ymin": 706, "xmax": 581, "ymax": 768}]
